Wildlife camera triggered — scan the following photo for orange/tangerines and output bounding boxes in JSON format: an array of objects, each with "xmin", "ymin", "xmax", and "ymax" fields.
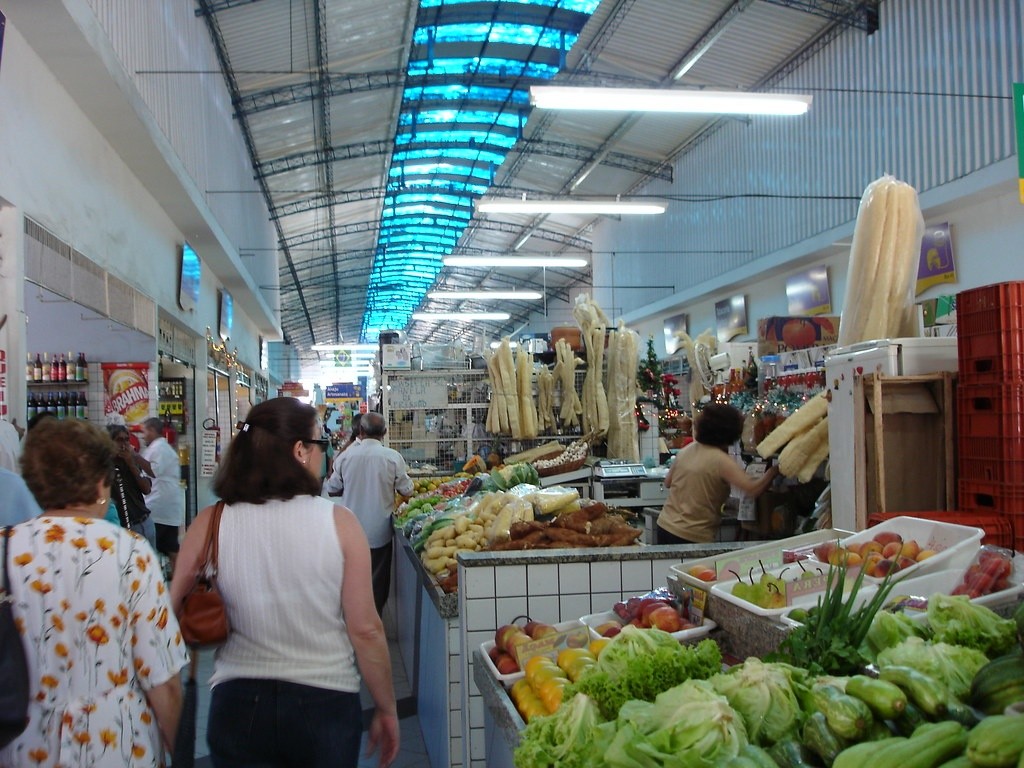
[
  {"xmin": 414, "ymin": 477, "xmax": 451, "ymax": 494},
  {"xmin": 781, "ymin": 319, "xmax": 817, "ymax": 347}
]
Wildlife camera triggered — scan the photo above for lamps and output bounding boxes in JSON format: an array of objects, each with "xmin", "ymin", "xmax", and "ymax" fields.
[
  {"xmin": 412, "ymin": 312, "xmax": 511, "ymax": 321},
  {"xmin": 442, "ymin": 253, "xmax": 591, "ymax": 269},
  {"xmin": 528, "ymin": 84, "xmax": 814, "ymax": 117},
  {"xmin": 428, "ymin": 289, "xmax": 544, "ymax": 300},
  {"xmin": 476, "ymin": 194, "xmax": 670, "ymax": 216}
]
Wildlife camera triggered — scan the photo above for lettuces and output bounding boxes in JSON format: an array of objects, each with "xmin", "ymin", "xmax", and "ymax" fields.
[
  {"xmin": 562, "ymin": 624, "xmax": 815, "ymax": 768},
  {"xmin": 869, "ymin": 592, "xmax": 1017, "ymax": 696},
  {"xmin": 514, "ymin": 692, "xmax": 611, "ymax": 768}
]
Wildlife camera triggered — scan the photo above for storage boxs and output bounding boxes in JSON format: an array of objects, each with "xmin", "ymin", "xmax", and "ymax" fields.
[{"xmin": 476, "ymin": 280, "xmax": 1024, "ymax": 686}]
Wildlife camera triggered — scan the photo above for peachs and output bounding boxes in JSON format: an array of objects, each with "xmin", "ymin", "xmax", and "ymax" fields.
[
  {"xmin": 952, "ymin": 550, "xmax": 1013, "ymax": 599},
  {"xmin": 813, "ymin": 532, "xmax": 938, "ymax": 578}
]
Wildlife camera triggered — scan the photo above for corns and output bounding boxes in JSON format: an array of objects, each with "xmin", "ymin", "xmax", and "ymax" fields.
[{"xmin": 479, "ymin": 490, "xmax": 579, "ymax": 540}]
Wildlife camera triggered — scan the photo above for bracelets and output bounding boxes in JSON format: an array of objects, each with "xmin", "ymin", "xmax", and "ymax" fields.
[{"xmin": 137, "ymin": 473, "xmax": 143, "ymax": 480}]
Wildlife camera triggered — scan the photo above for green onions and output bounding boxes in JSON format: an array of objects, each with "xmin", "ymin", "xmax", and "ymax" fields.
[{"xmin": 812, "ymin": 536, "xmax": 905, "ymax": 648}]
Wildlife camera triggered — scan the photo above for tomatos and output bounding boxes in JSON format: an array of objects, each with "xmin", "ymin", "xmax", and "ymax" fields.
[{"xmin": 446, "ymin": 479, "xmax": 471, "ymax": 497}]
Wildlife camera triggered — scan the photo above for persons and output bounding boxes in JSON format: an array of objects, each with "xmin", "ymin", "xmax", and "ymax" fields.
[
  {"xmin": 107, "ymin": 424, "xmax": 156, "ymax": 552},
  {"xmin": 319, "ymin": 413, "xmax": 364, "ymax": 501},
  {"xmin": 0, "ymin": 417, "xmax": 189, "ymax": 768},
  {"xmin": 0, "ymin": 418, "xmax": 40, "ymax": 528},
  {"xmin": 325, "ymin": 412, "xmax": 414, "ymax": 620},
  {"xmin": 136, "ymin": 418, "xmax": 182, "ymax": 578},
  {"xmin": 169, "ymin": 398, "xmax": 399, "ymax": 768},
  {"xmin": 657, "ymin": 402, "xmax": 780, "ymax": 544}
]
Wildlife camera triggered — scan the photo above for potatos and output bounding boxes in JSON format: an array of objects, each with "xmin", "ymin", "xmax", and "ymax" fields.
[{"xmin": 421, "ymin": 502, "xmax": 643, "ymax": 592}]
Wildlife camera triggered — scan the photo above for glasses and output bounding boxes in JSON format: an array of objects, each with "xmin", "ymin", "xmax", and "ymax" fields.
[{"xmin": 295, "ymin": 436, "xmax": 331, "ymax": 455}]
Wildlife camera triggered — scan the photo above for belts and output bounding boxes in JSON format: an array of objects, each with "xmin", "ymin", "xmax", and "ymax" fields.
[{"xmin": 131, "ymin": 513, "xmax": 150, "ymax": 525}]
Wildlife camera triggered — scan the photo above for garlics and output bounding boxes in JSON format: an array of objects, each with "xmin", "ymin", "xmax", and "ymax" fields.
[{"xmin": 532, "ymin": 441, "xmax": 588, "ymax": 468}]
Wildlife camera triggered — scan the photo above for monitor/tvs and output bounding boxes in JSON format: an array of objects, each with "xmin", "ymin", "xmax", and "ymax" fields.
[
  {"xmin": 260, "ymin": 338, "xmax": 268, "ymax": 370},
  {"xmin": 220, "ymin": 288, "xmax": 233, "ymax": 341},
  {"xmin": 180, "ymin": 239, "xmax": 202, "ymax": 312}
]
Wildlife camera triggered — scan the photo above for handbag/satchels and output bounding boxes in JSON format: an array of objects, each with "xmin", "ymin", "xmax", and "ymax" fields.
[
  {"xmin": 0, "ymin": 524, "xmax": 31, "ymax": 749},
  {"xmin": 178, "ymin": 498, "xmax": 231, "ymax": 647}
]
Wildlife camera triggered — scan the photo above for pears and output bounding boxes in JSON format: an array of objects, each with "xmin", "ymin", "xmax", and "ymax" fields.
[{"xmin": 728, "ymin": 560, "xmax": 822, "ymax": 610}]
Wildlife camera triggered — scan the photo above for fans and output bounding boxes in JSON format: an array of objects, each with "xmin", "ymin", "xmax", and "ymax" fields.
[{"xmin": 693, "ymin": 342, "xmax": 732, "ymax": 393}]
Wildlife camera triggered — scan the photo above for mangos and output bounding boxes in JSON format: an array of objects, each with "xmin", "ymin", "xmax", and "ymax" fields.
[{"xmin": 511, "ymin": 637, "xmax": 614, "ymax": 719}]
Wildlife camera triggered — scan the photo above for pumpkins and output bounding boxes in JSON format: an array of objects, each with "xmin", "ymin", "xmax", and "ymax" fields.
[{"xmin": 463, "ymin": 456, "xmax": 486, "ymax": 475}]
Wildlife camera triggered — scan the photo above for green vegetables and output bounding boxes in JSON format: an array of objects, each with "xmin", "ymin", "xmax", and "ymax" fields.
[
  {"xmin": 394, "ymin": 495, "xmax": 440, "ymax": 528},
  {"xmin": 763, "ymin": 625, "xmax": 863, "ymax": 678}
]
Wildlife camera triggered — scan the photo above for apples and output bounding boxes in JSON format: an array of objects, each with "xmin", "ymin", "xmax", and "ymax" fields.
[
  {"xmin": 688, "ymin": 565, "xmax": 719, "ymax": 582},
  {"xmin": 489, "ymin": 597, "xmax": 695, "ymax": 675}
]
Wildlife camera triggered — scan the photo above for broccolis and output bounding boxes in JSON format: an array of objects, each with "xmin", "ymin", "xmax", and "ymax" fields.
[{"xmin": 498, "ymin": 464, "xmax": 524, "ymax": 485}]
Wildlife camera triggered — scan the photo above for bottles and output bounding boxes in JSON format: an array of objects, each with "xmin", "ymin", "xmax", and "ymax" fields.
[
  {"xmin": 27, "ymin": 392, "xmax": 36, "ymax": 423},
  {"xmin": 37, "ymin": 392, "xmax": 46, "ymax": 414},
  {"xmin": 784, "ymin": 366, "xmax": 826, "ymax": 388},
  {"xmin": 48, "ymin": 392, "xmax": 56, "ymax": 417},
  {"xmin": 58, "ymin": 354, "xmax": 66, "ymax": 382},
  {"xmin": 77, "ymin": 392, "xmax": 89, "ymax": 419},
  {"xmin": 50, "ymin": 354, "xmax": 58, "ymax": 382},
  {"xmin": 177, "ymin": 437, "xmax": 189, "ymax": 485},
  {"xmin": 66, "ymin": 352, "xmax": 75, "ymax": 382},
  {"xmin": 159, "ymin": 382, "xmax": 182, "ymax": 395},
  {"xmin": 25, "ymin": 352, "xmax": 33, "ymax": 382},
  {"xmin": 75, "ymin": 352, "xmax": 88, "ymax": 381},
  {"xmin": 33, "ymin": 353, "xmax": 42, "ymax": 382},
  {"xmin": 42, "ymin": 353, "xmax": 51, "ymax": 382},
  {"xmin": 758, "ymin": 355, "xmax": 784, "ymax": 396},
  {"xmin": 68, "ymin": 392, "xmax": 76, "ymax": 417},
  {"xmin": 57, "ymin": 392, "xmax": 68, "ymax": 419}
]
